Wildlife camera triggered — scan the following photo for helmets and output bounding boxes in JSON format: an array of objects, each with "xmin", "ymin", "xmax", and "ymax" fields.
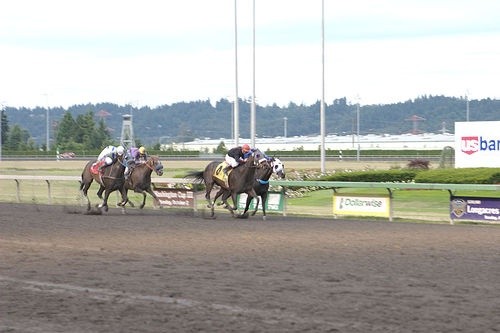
[
  {"xmin": 243, "ymin": 144, "xmax": 250, "ymax": 152},
  {"xmin": 117, "ymin": 146, "xmax": 124, "ymax": 156},
  {"xmin": 139, "ymin": 147, "xmax": 146, "ymax": 154}
]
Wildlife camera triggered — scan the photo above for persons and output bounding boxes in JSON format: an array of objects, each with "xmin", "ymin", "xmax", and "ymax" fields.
[
  {"xmin": 222, "ymin": 145, "xmax": 250, "ymax": 177},
  {"xmin": 97, "ymin": 145, "xmax": 124, "ymax": 171},
  {"xmin": 122, "ymin": 146, "xmax": 148, "ymax": 166},
  {"xmin": 243, "ymin": 148, "xmax": 272, "ymax": 162}
]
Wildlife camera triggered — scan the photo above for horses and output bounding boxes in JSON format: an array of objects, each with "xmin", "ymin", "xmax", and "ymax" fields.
[
  {"xmin": 80, "ymin": 156, "xmax": 164, "ymax": 215},
  {"xmin": 184, "ymin": 148, "xmax": 285, "ymax": 220}
]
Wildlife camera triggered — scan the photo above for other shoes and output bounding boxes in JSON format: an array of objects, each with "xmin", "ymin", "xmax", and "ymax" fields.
[{"xmin": 98, "ymin": 166, "xmax": 103, "ymax": 171}]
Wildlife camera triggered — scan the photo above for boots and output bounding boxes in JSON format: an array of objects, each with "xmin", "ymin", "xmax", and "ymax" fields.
[{"xmin": 223, "ymin": 165, "xmax": 232, "ymax": 174}]
